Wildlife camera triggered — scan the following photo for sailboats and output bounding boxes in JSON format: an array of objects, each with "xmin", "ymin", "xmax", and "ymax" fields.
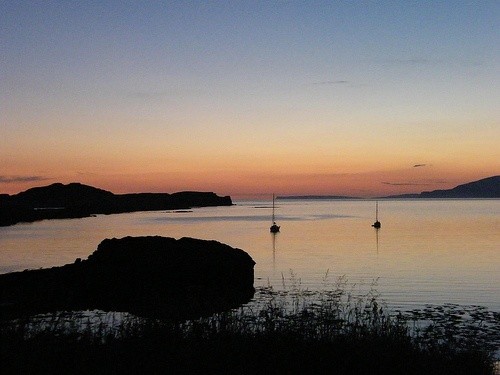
[
  {"xmin": 373, "ymin": 200, "xmax": 382, "ymax": 229},
  {"xmin": 269, "ymin": 195, "xmax": 280, "ymax": 234}
]
